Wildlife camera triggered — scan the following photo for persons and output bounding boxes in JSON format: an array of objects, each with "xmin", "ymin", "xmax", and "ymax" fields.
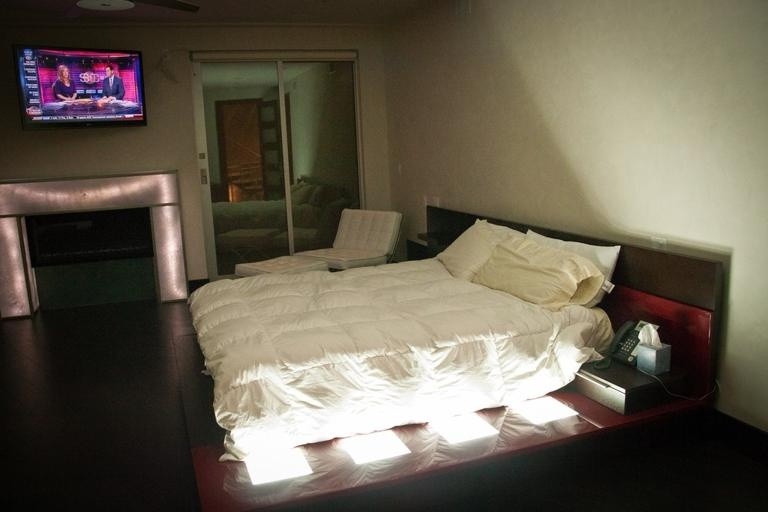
[
  {"xmin": 101, "ymin": 65, "xmax": 125, "ymax": 103},
  {"xmin": 55, "ymin": 64, "xmax": 78, "ymax": 102}
]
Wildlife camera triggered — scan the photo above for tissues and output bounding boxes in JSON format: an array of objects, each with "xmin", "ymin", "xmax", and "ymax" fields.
[{"xmin": 636, "ymin": 322, "xmax": 673, "ymax": 376}]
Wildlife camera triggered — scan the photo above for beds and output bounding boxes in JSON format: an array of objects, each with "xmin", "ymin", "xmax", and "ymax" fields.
[
  {"xmin": 212, "ymin": 174, "xmax": 346, "ymax": 234},
  {"xmin": 172, "ymin": 206, "xmax": 723, "ymax": 510}
]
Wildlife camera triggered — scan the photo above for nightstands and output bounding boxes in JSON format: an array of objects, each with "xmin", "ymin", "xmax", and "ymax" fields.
[{"xmin": 405, "ymin": 238, "xmax": 444, "ymax": 259}]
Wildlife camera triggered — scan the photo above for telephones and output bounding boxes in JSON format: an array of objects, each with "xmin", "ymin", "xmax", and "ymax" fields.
[{"xmin": 603, "ymin": 319, "xmax": 663, "ymax": 365}]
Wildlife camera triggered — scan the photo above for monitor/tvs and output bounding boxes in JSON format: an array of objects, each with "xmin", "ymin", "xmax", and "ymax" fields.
[{"xmin": 12, "ymin": 43, "xmax": 147, "ymax": 130}]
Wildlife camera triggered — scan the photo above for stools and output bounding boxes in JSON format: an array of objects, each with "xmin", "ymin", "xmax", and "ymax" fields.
[
  {"xmin": 234, "ymin": 255, "xmax": 329, "ymax": 277},
  {"xmin": 214, "ymin": 227, "xmax": 279, "ymax": 263}
]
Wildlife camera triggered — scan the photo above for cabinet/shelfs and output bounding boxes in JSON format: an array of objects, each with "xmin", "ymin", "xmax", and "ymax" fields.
[{"xmin": 0, "ymin": 169, "xmax": 193, "ymax": 322}]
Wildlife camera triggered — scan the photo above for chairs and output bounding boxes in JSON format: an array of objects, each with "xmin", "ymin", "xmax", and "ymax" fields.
[
  {"xmin": 290, "ymin": 207, "xmax": 403, "ymax": 270},
  {"xmin": 274, "ymin": 199, "xmax": 349, "ymax": 254}
]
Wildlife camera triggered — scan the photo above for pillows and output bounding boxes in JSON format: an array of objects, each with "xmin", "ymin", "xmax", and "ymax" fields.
[
  {"xmin": 434, "ymin": 219, "xmax": 499, "ymax": 280},
  {"xmin": 470, "ymin": 234, "xmax": 601, "ymax": 312},
  {"xmin": 525, "ymin": 227, "xmax": 622, "ymax": 312}
]
